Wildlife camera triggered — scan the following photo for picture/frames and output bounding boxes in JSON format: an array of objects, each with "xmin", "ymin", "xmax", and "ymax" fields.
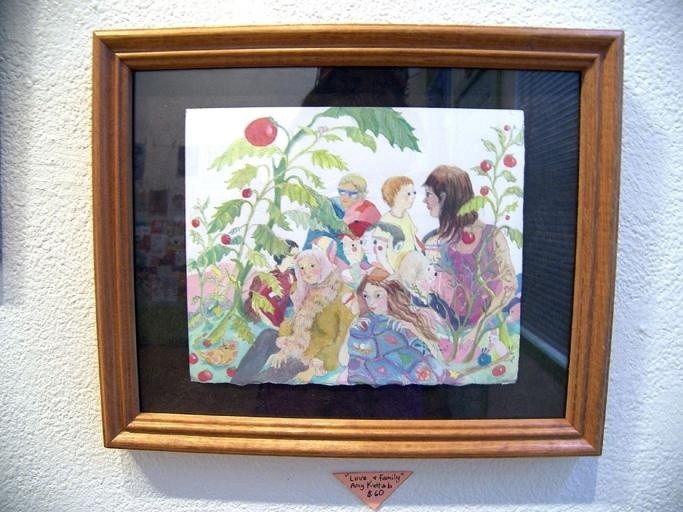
[{"xmin": 90, "ymin": 21, "xmax": 623, "ymax": 459}]
